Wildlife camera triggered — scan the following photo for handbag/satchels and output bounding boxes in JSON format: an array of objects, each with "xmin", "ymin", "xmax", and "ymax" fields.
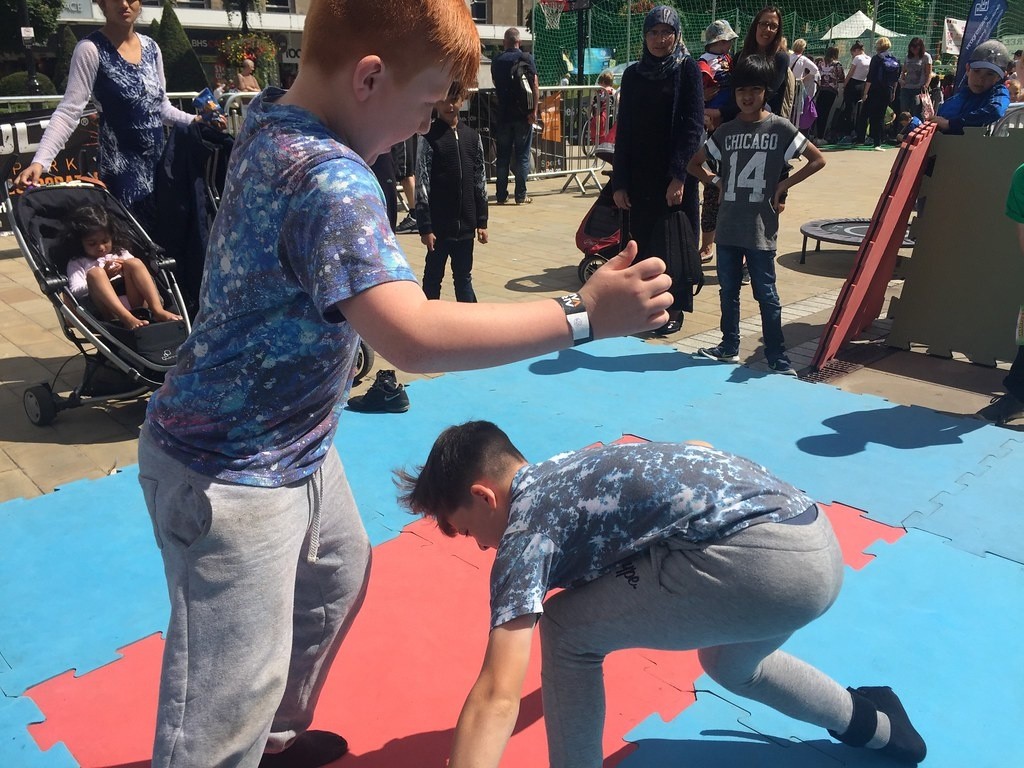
[
  {"xmin": 799, "ymin": 95, "xmax": 818, "ymax": 129},
  {"xmin": 919, "ymin": 88, "xmax": 935, "ymax": 120}
]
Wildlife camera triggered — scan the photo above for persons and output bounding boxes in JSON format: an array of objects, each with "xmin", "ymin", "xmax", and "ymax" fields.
[
  {"xmin": 236, "ymin": 59, "xmax": 261, "ymax": 91},
  {"xmin": 930, "ymin": 73, "xmax": 954, "ymax": 108},
  {"xmin": 1003, "ymin": 50, "xmax": 1024, "ymax": 102},
  {"xmin": 137, "ymin": 0, "xmax": 675, "ymax": 768},
  {"xmin": 214, "ymin": 78, "xmax": 235, "ymax": 115},
  {"xmin": 392, "ymin": 420, "xmax": 928, "ymax": 768},
  {"xmin": 968, "ymin": 185, "xmax": 1024, "ymax": 423},
  {"xmin": 414, "ymin": 81, "xmax": 488, "ymax": 304},
  {"xmin": 66, "ymin": 204, "xmax": 183, "ymax": 330},
  {"xmin": 591, "ymin": 71, "xmax": 620, "ymax": 130},
  {"xmin": 788, "ymin": 37, "xmax": 933, "ymax": 147},
  {"xmin": 491, "ymin": 28, "xmax": 540, "ymax": 206},
  {"xmin": 612, "ymin": 5, "xmax": 705, "ymax": 332},
  {"xmin": 927, "ymin": 40, "xmax": 1010, "ymax": 136},
  {"xmin": 697, "ymin": 6, "xmax": 795, "ymax": 137},
  {"xmin": 13, "ymin": 0, "xmax": 203, "ymax": 188},
  {"xmin": 561, "ymin": 73, "xmax": 570, "ymax": 86},
  {"xmin": 687, "ymin": 55, "xmax": 827, "ymax": 377},
  {"xmin": 372, "ymin": 134, "xmax": 418, "ymax": 233}
]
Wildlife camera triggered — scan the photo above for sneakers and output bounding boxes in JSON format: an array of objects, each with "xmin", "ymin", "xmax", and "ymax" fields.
[
  {"xmin": 516, "ymin": 194, "xmax": 534, "ymax": 205},
  {"xmin": 347, "ymin": 370, "xmax": 410, "ymax": 413},
  {"xmin": 395, "ymin": 214, "xmax": 419, "ymax": 234},
  {"xmin": 497, "ymin": 196, "xmax": 509, "ymax": 204},
  {"xmin": 767, "ymin": 355, "xmax": 797, "ymax": 375},
  {"xmin": 973, "ymin": 393, "xmax": 1024, "ymax": 424},
  {"xmin": 698, "ymin": 343, "xmax": 740, "ymax": 363}
]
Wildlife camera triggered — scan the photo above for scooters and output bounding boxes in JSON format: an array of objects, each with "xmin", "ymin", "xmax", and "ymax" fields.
[{"xmin": 575, "ymin": 147, "xmax": 628, "ymax": 285}]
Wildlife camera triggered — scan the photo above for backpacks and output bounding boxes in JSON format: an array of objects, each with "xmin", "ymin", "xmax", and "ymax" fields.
[{"xmin": 877, "ymin": 53, "xmax": 900, "ymax": 87}]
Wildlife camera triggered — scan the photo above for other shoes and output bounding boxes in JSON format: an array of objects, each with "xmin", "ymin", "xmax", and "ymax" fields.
[
  {"xmin": 655, "ymin": 311, "xmax": 683, "ymax": 333},
  {"xmin": 826, "ymin": 686, "xmax": 927, "ymax": 765},
  {"xmin": 257, "ymin": 729, "xmax": 346, "ymax": 768},
  {"xmin": 699, "ymin": 251, "xmax": 713, "ymax": 263},
  {"xmin": 814, "ymin": 137, "xmax": 828, "ymax": 145},
  {"xmin": 863, "ymin": 137, "xmax": 875, "ymax": 145},
  {"xmin": 741, "ymin": 263, "xmax": 751, "ymax": 284},
  {"xmin": 837, "ymin": 137, "xmax": 852, "ymax": 145},
  {"xmin": 875, "ymin": 146, "xmax": 881, "ymax": 150}
]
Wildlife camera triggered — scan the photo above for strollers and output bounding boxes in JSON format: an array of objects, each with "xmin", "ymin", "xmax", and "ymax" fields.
[{"xmin": 2, "ymin": 175, "xmax": 192, "ymax": 428}]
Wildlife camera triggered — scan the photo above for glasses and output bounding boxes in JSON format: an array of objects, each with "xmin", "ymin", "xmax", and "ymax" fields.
[
  {"xmin": 757, "ymin": 21, "xmax": 779, "ymax": 33},
  {"xmin": 647, "ymin": 29, "xmax": 675, "ymax": 37}
]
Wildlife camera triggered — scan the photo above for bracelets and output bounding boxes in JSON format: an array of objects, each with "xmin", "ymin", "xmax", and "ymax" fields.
[{"xmin": 555, "ymin": 293, "xmax": 594, "ymax": 347}]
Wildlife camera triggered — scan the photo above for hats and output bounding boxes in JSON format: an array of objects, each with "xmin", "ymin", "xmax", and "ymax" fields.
[
  {"xmin": 704, "ymin": 20, "xmax": 739, "ymax": 46},
  {"xmin": 1011, "ymin": 50, "xmax": 1023, "ymax": 56},
  {"xmin": 970, "ymin": 39, "xmax": 1009, "ymax": 80}
]
